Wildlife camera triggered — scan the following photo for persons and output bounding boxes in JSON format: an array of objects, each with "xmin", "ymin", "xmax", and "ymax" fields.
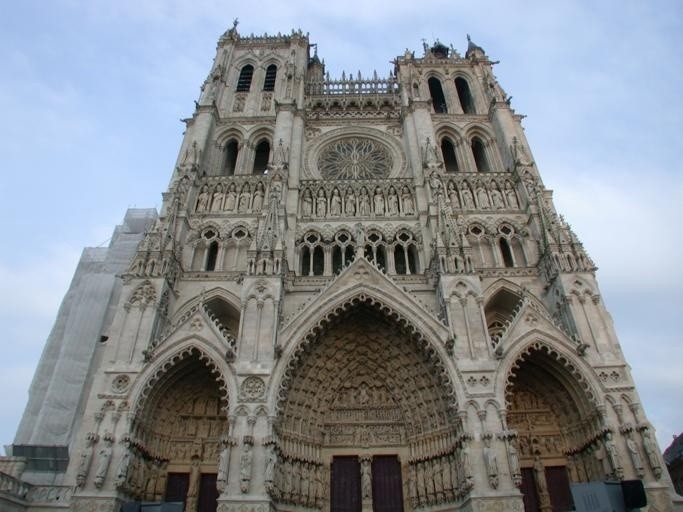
[
  {"xmin": 188, "ymin": 462, "xmax": 201, "ymax": 497},
  {"xmin": 361, "ymin": 461, "xmax": 371, "ymax": 499},
  {"xmin": 77, "ymin": 439, "xmax": 169, "ymax": 493},
  {"xmin": 301, "ymin": 186, "xmax": 413, "ymax": 217},
  {"xmin": 217, "ymin": 444, "xmax": 325, "ymax": 501},
  {"xmin": 407, "ymin": 442, "xmax": 473, "ymax": 503},
  {"xmin": 566, "ymin": 429, "xmax": 663, "ymax": 482},
  {"xmin": 352, "ymin": 380, "xmax": 380, "ymax": 404},
  {"xmin": 481, "ymin": 440, "xmax": 521, "ymax": 477},
  {"xmin": 448, "ymin": 181, "xmax": 519, "ymax": 209},
  {"xmin": 534, "ymin": 456, "xmax": 546, "ymax": 492},
  {"xmin": 197, "ymin": 182, "xmax": 265, "ymax": 212}
]
